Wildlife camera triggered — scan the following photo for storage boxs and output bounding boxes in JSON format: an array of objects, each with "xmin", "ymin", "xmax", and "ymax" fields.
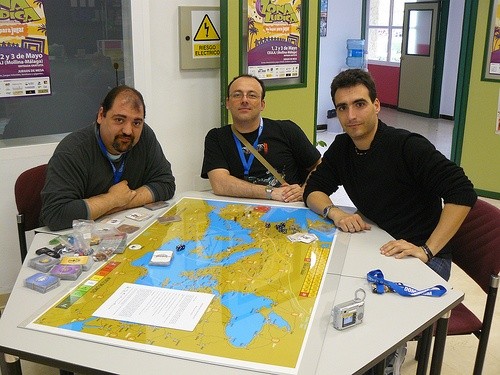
[
  {"xmin": 24, "ymin": 233, "xmax": 127, "ymax": 294},
  {"xmin": 149, "ymin": 250, "xmax": 174, "ymax": 266}
]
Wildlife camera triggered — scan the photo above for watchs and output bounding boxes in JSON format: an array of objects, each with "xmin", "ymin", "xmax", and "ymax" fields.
[
  {"xmin": 323, "ymin": 205, "xmax": 338, "ymax": 218},
  {"xmin": 265, "ymin": 186, "xmax": 273, "ymax": 200}
]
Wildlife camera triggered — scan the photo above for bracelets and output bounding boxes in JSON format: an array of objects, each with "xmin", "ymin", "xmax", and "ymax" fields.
[{"xmin": 422, "ymin": 244, "xmax": 433, "ymax": 260}]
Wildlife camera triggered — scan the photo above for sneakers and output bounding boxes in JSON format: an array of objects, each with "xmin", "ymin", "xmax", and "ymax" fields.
[{"xmin": 382, "ymin": 341, "xmax": 407, "ymax": 375}]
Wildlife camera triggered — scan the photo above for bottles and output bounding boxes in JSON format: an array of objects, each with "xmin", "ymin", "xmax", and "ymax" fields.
[{"xmin": 346, "ymin": 39, "xmax": 367, "ymax": 68}]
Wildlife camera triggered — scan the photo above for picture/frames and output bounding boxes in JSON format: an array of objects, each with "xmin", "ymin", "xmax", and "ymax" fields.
[
  {"xmin": 480, "ymin": 0, "xmax": 500, "ymax": 83},
  {"xmin": 239, "ymin": 0, "xmax": 309, "ymax": 91}
]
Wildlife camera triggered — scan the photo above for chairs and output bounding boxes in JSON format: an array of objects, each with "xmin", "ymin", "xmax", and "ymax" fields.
[
  {"xmin": 14, "ymin": 163, "xmax": 49, "ymax": 264},
  {"xmin": 408, "ymin": 199, "xmax": 500, "ymax": 375}
]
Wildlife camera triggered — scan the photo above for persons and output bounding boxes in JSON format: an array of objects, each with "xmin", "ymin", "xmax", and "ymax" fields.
[
  {"xmin": 201, "ymin": 74, "xmax": 322, "ymax": 203},
  {"xmin": 303, "ymin": 68, "xmax": 478, "ymax": 375},
  {"xmin": 40, "ymin": 85, "xmax": 176, "ymax": 232}
]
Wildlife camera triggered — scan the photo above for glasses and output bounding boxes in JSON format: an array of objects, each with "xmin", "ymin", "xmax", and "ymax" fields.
[{"xmin": 229, "ymin": 92, "xmax": 262, "ymax": 102}]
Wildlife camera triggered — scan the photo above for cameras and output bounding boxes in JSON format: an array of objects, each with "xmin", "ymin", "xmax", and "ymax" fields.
[{"xmin": 332, "ymin": 298, "xmax": 364, "ymax": 330}]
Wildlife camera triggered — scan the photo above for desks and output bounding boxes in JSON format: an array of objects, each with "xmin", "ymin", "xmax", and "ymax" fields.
[{"xmin": 0, "ymin": 191, "xmax": 465, "ymax": 375}]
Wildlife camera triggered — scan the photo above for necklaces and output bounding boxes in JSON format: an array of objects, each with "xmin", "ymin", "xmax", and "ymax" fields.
[{"xmin": 354, "ymin": 146, "xmax": 369, "ymax": 156}]
